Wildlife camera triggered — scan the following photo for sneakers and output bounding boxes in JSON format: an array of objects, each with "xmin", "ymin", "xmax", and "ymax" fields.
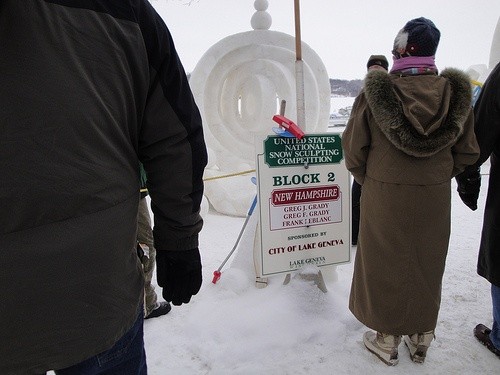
[{"xmin": 144, "ymin": 302, "xmax": 171, "ymax": 319}]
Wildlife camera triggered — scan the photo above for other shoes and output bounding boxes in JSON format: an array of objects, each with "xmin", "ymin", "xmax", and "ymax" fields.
[
  {"xmin": 352, "ymin": 241, "xmax": 357, "ymax": 245},
  {"xmin": 474, "ymin": 324, "xmax": 500, "ymax": 356}
]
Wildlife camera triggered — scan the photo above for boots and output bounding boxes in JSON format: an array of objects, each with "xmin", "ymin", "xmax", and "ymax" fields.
[
  {"xmin": 404, "ymin": 330, "xmax": 435, "ymax": 363},
  {"xmin": 363, "ymin": 330, "xmax": 401, "ymax": 366}
]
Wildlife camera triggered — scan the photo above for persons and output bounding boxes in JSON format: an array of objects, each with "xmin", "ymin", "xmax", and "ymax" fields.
[
  {"xmin": 455, "ymin": 62, "xmax": 500, "ymax": 359},
  {"xmin": 138, "ymin": 164, "xmax": 171, "ymax": 320},
  {"xmin": 342, "ymin": 17, "xmax": 481, "ymax": 366},
  {"xmin": 352, "ymin": 55, "xmax": 389, "ymax": 248},
  {"xmin": 0, "ymin": 0, "xmax": 207, "ymax": 375}
]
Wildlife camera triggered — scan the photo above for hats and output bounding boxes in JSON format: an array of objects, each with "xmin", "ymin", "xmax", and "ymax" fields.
[
  {"xmin": 392, "ymin": 17, "xmax": 441, "ymax": 59},
  {"xmin": 367, "ymin": 55, "xmax": 389, "ymax": 72}
]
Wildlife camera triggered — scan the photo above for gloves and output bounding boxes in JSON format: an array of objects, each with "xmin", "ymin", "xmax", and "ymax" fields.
[
  {"xmin": 156, "ymin": 247, "xmax": 202, "ymax": 306},
  {"xmin": 457, "ymin": 170, "xmax": 482, "ymax": 211}
]
